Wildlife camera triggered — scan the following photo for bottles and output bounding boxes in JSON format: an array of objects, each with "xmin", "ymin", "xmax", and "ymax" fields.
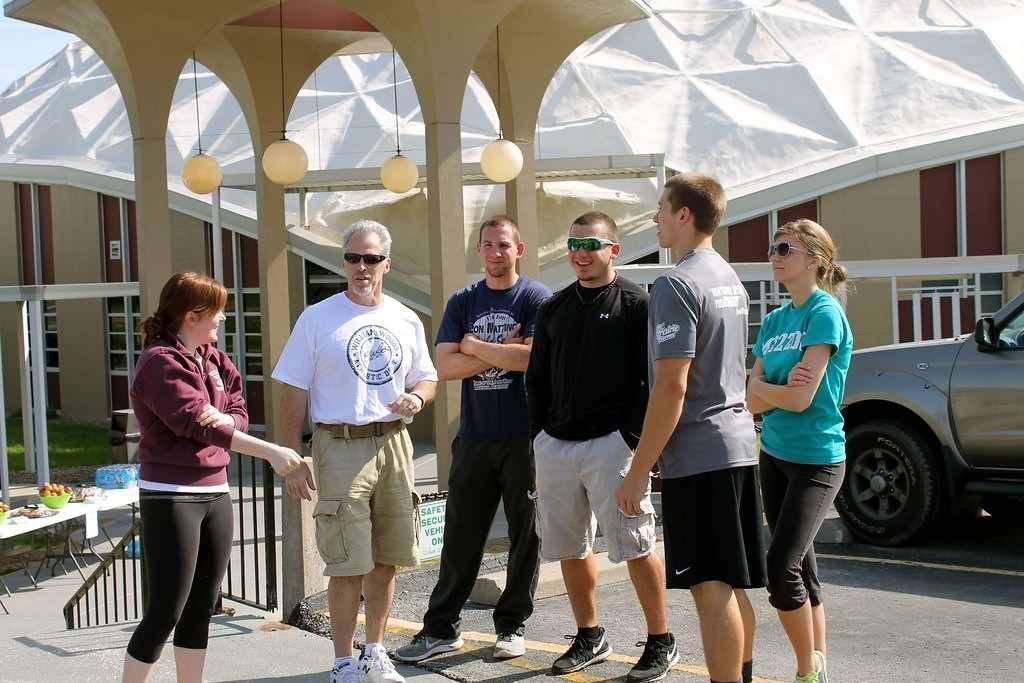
[
  {"xmin": 74, "ymin": 483, "xmax": 86, "ymax": 502},
  {"xmin": 96, "ymin": 463, "xmax": 141, "ymax": 488},
  {"xmin": 127, "ymin": 535, "xmax": 140, "ymax": 558}
]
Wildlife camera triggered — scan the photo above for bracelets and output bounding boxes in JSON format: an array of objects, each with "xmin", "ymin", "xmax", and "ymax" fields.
[{"xmin": 409, "ymin": 391, "xmax": 426, "ymax": 410}]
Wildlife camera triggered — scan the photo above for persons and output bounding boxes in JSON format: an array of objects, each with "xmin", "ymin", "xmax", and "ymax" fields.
[
  {"xmin": 390, "ymin": 173, "xmax": 774, "ymax": 683},
  {"xmin": 120, "ymin": 273, "xmax": 307, "ymax": 683},
  {"xmin": 746, "ymin": 216, "xmax": 853, "ymax": 683},
  {"xmin": 271, "ymin": 220, "xmax": 438, "ymax": 683}
]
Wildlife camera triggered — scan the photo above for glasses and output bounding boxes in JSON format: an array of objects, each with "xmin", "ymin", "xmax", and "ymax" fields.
[
  {"xmin": 566, "ymin": 236, "xmax": 616, "ymax": 252},
  {"xmin": 344, "ymin": 252, "xmax": 386, "ymax": 265},
  {"xmin": 767, "ymin": 241, "xmax": 814, "ymax": 259}
]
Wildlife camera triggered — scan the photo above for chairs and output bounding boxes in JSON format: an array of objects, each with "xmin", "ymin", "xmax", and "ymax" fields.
[
  {"xmin": 54, "ymin": 522, "xmax": 89, "ymax": 569},
  {"xmin": 73, "ymin": 516, "xmax": 117, "ymax": 550},
  {"xmin": 23, "ymin": 531, "xmax": 65, "ymax": 576}
]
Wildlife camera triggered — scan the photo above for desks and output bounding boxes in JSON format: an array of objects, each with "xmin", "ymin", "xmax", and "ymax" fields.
[
  {"xmin": 50, "ymin": 486, "xmax": 140, "ymax": 577},
  {"xmin": 0, "ymin": 503, "xmax": 99, "ymax": 615}
]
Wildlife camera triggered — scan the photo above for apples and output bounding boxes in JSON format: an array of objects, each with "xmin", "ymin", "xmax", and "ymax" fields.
[
  {"xmin": 0, "ymin": 502, "xmax": 8, "ymax": 512},
  {"xmin": 39, "ymin": 482, "xmax": 72, "ymax": 496}
]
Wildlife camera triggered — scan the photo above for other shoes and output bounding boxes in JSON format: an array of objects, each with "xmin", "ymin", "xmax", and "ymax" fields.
[
  {"xmin": 813, "ymin": 651, "xmax": 828, "ymax": 683},
  {"xmin": 793, "ymin": 670, "xmax": 819, "ymax": 683}
]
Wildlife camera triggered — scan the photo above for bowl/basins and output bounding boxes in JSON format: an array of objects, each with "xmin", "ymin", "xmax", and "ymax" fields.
[
  {"xmin": 37, "ymin": 491, "xmax": 73, "ymax": 509},
  {"xmin": 0, "ymin": 510, "xmax": 8, "ymax": 525}
]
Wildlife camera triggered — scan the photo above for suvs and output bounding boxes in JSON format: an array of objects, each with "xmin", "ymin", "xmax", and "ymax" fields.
[{"xmin": 834, "ymin": 286, "xmax": 1024, "ymax": 549}]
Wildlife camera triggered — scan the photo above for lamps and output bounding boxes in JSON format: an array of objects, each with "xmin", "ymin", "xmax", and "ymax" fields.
[
  {"xmin": 261, "ymin": 0, "xmax": 308, "ymax": 186},
  {"xmin": 380, "ymin": 47, "xmax": 419, "ymax": 193},
  {"xmin": 480, "ymin": 26, "xmax": 524, "ymax": 183},
  {"xmin": 181, "ymin": 50, "xmax": 223, "ymax": 195}
]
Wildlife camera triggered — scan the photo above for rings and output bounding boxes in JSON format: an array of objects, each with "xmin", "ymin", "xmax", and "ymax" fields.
[{"xmin": 408, "ymin": 405, "xmax": 413, "ymax": 409}]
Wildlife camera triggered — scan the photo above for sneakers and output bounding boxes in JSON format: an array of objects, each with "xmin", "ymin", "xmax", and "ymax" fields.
[
  {"xmin": 627, "ymin": 632, "xmax": 680, "ymax": 683},
  {"xmin": 357, "ymin": 645, "xmax": 407, "ymax": 683},
  {"xmin": 551, "ymin": 626, "xmax": 613, "ymax": 675},
  {"xmin": 393, "ymin": 630, "xmax": 465, "ymax": 662},
  {"xmin": 493, "ymin": 632, "xmax": 526, "ymax": 657},
  {"xmin": 330, "ymin": 657, "xmax": 364, "ymax": 682}
]
